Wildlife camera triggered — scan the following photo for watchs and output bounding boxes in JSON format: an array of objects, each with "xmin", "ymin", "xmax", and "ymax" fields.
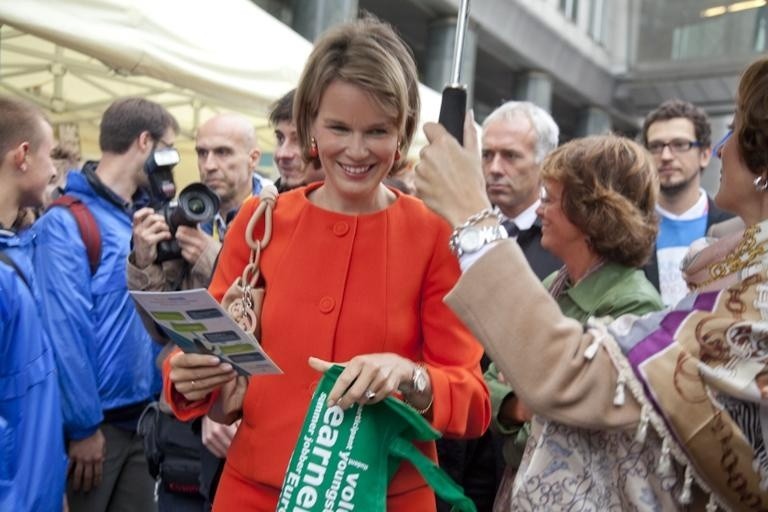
[
  {"xmin": 404, "ymin": 362, "xmax": 430, "ymax": 405},
  {"xmin": 406, "ymin": 365, "xmax": 434, "ymax": 417},
  {"xmin": 449, "ymin": 223, "xmax": 507, "ymax": 258}
]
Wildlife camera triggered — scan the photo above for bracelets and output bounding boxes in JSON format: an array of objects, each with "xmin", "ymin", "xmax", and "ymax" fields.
[{"xmin": 449, "ymin": 205, "xmax": 506, "ymax": 239}]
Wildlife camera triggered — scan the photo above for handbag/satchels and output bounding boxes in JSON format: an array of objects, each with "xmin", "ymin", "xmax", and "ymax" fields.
[
  {"xmin": 207, "ymin": 185, "xmax": 280, "ymax": 426},
  {"xmin": 275, "ymin": 364, "xmax": 477, "ymax": 512},
  {"xmin": 136, "ymin": 402, "xmax": 200, "ymax": 494}
]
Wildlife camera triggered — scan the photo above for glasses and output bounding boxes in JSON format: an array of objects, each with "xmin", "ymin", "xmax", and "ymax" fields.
[{"xmin": 647, "ymin": 137, "xmax": 704, "ymax": 154}]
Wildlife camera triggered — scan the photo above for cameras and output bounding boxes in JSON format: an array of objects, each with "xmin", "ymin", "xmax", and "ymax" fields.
[{"xmin": 145, "ymin": 148, "xmax": 221, "ymax": 264}]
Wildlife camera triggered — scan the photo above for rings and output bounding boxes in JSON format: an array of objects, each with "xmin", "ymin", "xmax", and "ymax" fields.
[
  {"xmin": 365, "ymin": 390, "xmax": 377, "ymax": 401},
  {"xmin": 189, "ymin": 377, "xmax": 195, "ymax": 390}
]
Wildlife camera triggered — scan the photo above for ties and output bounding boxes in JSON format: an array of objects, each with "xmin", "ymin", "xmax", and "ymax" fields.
[{"xmin": 500, "ymin": 219, "xmax": 520, "ymax": 237}]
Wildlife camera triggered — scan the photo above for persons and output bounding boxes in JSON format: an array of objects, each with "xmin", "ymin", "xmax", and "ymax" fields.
[
  {"xmin": 23, "ymin": 147, "xmax": 80, "ymax": 233},
  {"xmin": 479, "ymin": 133, "xmax": 666, "ymax": 464},
  {"xmin": 640, "ymin": 97, "xmax": 733, "ymax": 308},
  {"xmin": 270, "ymin": 86, "xmax": 323, "ymax": 197},
  {"xmin": 127, "ymin": 109, "xmax": 275, "ymax": 340},
  {"xmin": 477, "ymin": 101, "xmax": 566, "ymax": 284},
  {"xmin": 162, "ymin": 17, "xmax": 493, "ymax": 512},
  {"xmin": 34, "ymin": 94, "xmax": 183, "ymax": 511},
  {"xmin": 0, "ymin": 96, "xmax": 68, "ymax": 511},
  {"xmin": 414, "ymin": 60, "xmax": 766, "ymax": 512}
]
